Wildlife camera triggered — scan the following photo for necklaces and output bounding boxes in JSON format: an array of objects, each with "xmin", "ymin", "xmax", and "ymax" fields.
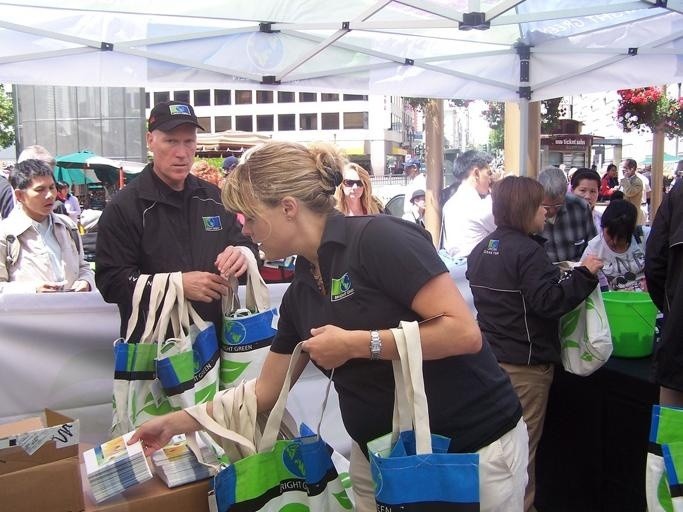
[{"xmin": 313, "ymin": 272, "xmax": 323, "ymax": 291}]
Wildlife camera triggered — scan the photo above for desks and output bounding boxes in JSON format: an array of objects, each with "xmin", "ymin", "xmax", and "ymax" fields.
[{"xmin": 534, "ymin": 356, "xmax": 658, "ymax": 512}]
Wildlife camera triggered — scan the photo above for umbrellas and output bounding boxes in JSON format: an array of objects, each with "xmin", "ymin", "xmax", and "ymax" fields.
[{"xmin": 54, "ymin": 150, "xmax": 120, "ymax": 184}]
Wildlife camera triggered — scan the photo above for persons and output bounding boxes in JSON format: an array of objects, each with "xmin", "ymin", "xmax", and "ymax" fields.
[
  {"xmin": 641, "ymin": 168, "xmax": 682, "ymax": 410},
  {"xmin": 127, "ymin": 135, "xmax": 528, "ymax": 511},
  {"xmin": 388, "ymin": 146, "xmax": 682, "ymax": 262},
  {"xmin": 93, "ymin": 98, "xmax": 261, "ymax": 347},
  {"xmin": 0, "ymin": 142, "xmax": 93, "ymax": 294},
  {"xmin": 464, "ymin": 174, "xmax": 606, "ymax": 511},
  {"xmin": 577, "ymin": 198, "xmax": 654, "ymax": 295},
  {"xmin": 328, "ymin": 161, "xmax": 391, "ymax": 216}
]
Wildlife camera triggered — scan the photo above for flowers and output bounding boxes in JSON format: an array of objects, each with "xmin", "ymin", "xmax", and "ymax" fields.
[
  {"xmin": 448, "ymin": 99, "xmax": 474, "ymax": 108},
  {"xmin": 612, "ymin": 86, "xmax": 683, "ymax": 142},
  {"xmin": 402, "ymin": 97, "xmax": 429, "ymax": 112},
  {"xmin": 480, "ymin": 100, "xmax": 505, "ymax": 128}
]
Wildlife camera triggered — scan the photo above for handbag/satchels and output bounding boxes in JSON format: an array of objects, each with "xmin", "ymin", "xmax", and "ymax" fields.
[
  {"xmin": 366, "ymin": 429, "xmax": 481, "ymax": 512},
  {"xmin": 207, "ymin": 432, "xmax": 355, "ymax": 512},
  {"xmin": 552, "ymin": 260, "xmax": 614, "ymax": 377},
  {"xmin": 644, "ymin": 404, "xmax": 683, "ymax": 512},
  {"xmin": 111, "ymin": 339, "xmax": 173, "ymax": 438},
  {"xmin": 219, "ymin": 303, "xmax": 280, "ymax": 390},
  {"xmin": 153, "ymin": 320, "xmax": 220, "ymax": 414},
  {"xmin": 436, "ymin": 247, "xmax": 478, "ymax": 322}
]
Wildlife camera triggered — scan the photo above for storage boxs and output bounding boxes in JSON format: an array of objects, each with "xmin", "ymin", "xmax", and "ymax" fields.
[
  {"xmin": 0, "ymin": 408, "xmax": 85, "ymax": 512},
  {"xmin": 80, "ymin": 455, "xmax": 213, "ymax": 512}
]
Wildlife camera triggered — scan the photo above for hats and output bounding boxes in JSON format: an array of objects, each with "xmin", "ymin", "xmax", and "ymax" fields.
[
  {"xmin": 222, "ymin": 156, "xmax": 240, "ymax": 168},
  {"xmin": 400, "ymin": 158, "xmax": 421, "ymax": 168},
  {"xmin": 148, "ymin": 100, "xmax": 206, "ymax": 133},
  {"xmin": 410, "ymin": 189, "xmax": 425, "ymax": 202}
]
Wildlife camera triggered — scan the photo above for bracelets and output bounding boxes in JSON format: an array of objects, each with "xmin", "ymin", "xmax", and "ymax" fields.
[{"xmin": 369, "ymin": 330, "xmax": 382, "ymax": 361}]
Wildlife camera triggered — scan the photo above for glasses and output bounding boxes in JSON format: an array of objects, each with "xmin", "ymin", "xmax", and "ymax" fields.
[{"xmin": 343, "ymin": 179, "xmax": 365, "ymax": 188}]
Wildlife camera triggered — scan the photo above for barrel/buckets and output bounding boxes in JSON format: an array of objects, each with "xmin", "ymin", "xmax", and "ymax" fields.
[{"xmin": 601, "ymin": 291, "xmax": 660, "ymax": 357}]
